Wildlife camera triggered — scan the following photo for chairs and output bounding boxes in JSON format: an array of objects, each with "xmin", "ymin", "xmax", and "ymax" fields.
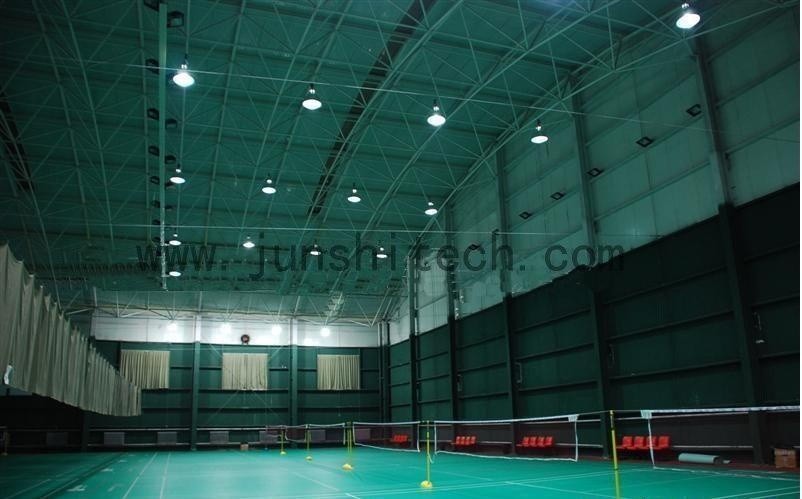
[{"xmin": 391, "ymin": 435, "xmax": 670, "ymax": 461}]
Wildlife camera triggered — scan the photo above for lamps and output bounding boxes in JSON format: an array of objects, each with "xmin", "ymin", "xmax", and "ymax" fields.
[{"xmin": 143, "ymin": 0, "xmax": 700, "ymax": 278}]
